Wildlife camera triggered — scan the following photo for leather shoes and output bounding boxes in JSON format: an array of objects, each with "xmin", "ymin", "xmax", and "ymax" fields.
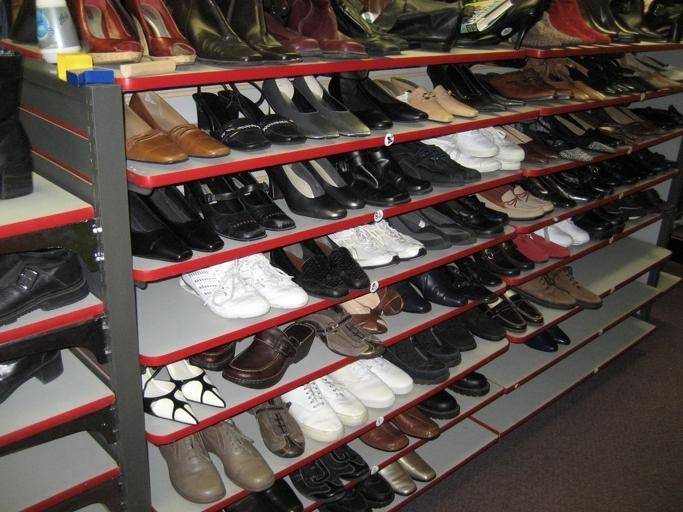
[
  {"xmin": 390, "ymin": 407, "xmax": 440, "ymax": 440},
  {"xmin": 226, "ymin": 492, "xmax": 272, "ymax": 511},
  {"xmin": 255, "ymin": 476, "xmax": 303, "ymax": 511},
  {"xmin": 159, "ymin": 432, "xmax": 226, "ymax": 504},
  {"xmin": 1, "ymin": 246, "xmax": 89, "ymax": 325},
  {"xmin": 359, "ymin": 421, "xmax": 409, "ymax": 452},
  {"xmin": 446, "ymin": 372, "xmax": 490, "ymax": 397},
  {"xmin": 398, "ymin": 450, "xmax": 436, "ymax": 482},
  {"xmin": 199, "ymin": 417, "xmax": 275, "ymax": 492},
  {"xmin": 378, "ymin": 462, "xmax": 417, "ymax": 496},
  {"xmin": 417, "ymin": 390, "xmax": 460, "ymax": 420},
  {"xmin": 77, "ymin": 1, "xmax": 678, "ymax": 385}
]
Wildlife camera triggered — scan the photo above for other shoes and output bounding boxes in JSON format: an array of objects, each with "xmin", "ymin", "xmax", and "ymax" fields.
[
  {"xmin": 317, "ymin": 488, "xmax": 372, "ymax": 510},
  {"xmin": 355, "ymin": 471, "xmax": 394, "ymax": 509},
  {"xmin": 290, "ymin": 458, "xmax": 347, "ymax": 503},
  {"xmin": 322, "ymin": 443, "xmax": 371, "ymax": 481},
  {"xmin": 246, "ymin": 392, "xmax": 305, "ymax": 459}
]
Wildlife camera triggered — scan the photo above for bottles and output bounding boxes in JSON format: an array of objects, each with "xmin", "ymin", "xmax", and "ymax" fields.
[{"xmin": 34, "ymin": 0, "xmax": 81, "ymax": 66}]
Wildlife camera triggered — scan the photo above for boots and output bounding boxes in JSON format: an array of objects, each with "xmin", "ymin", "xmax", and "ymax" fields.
[{"xmin": 2, "ymin": 50, "xmax": 34, "ymax": 200}]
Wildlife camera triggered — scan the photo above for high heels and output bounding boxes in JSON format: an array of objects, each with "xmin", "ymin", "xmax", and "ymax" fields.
[
  {"xmin": 141, "ymin": 365, "xmax": 199, "ymax": 426},
  {"xmin": 152, "ymin": 353, "xmax": 226, "ymax": 408}
]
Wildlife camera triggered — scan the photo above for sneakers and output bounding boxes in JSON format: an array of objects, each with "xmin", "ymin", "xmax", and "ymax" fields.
[
  {"xmin": 359, "ymin": 357, "xmax": 414, "ymax": 396},
  {"xmin": 329, "ymin": 362, "xmax": 395, "ymax": 409},
  {"xmin": 280, "ymin": 381, "xmax": 345, "ymax": 442},
  {"xmin": 313, "ymin": 375, "xmax": 368, "ymax": 428}
]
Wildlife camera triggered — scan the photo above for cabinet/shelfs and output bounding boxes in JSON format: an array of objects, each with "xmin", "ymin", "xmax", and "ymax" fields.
[
  {"xmin": 119, "ymin": 36, "xmax": 682, "ymax": 512},
  {"xmin": 0, "ymin": 170, "xmax": 121, "ymax": 512}
]
